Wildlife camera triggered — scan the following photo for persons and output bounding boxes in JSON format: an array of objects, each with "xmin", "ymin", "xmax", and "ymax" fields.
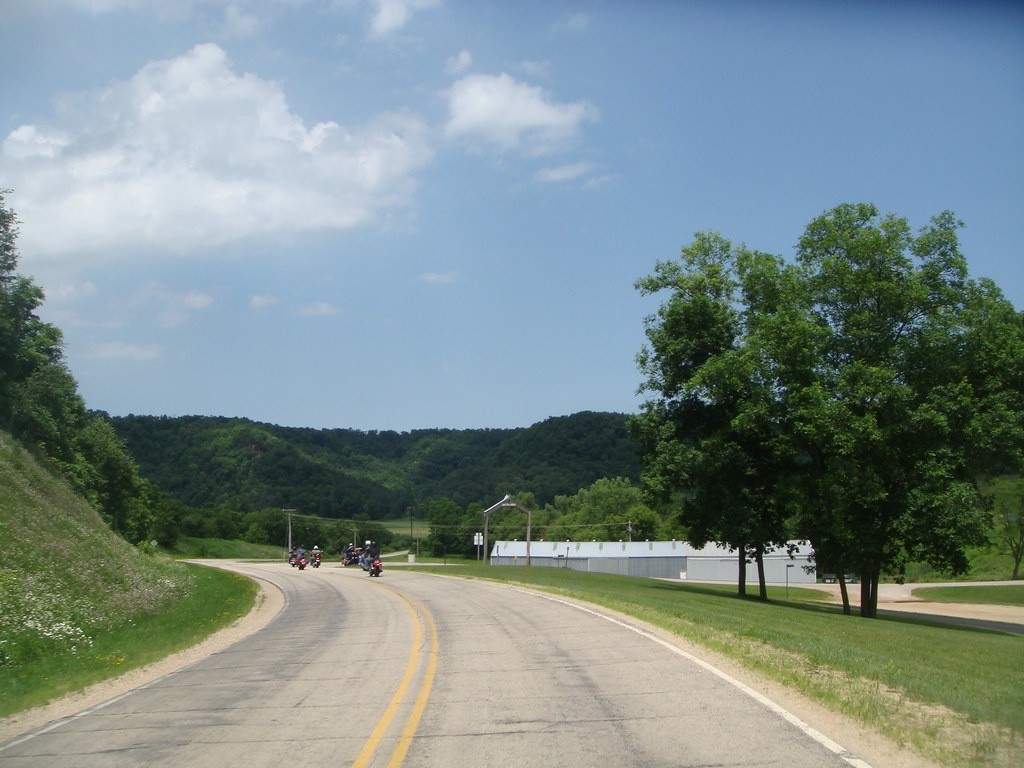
[
  {"xmin": 347, "ymin": 539, "xmax": 378, "ymax": 571},
  {"xmin": 289, "ymin": 544, "xmax": 321, "ymax": 565}
]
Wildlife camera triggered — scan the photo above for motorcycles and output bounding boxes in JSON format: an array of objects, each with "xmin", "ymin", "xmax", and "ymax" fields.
[
  {"xmin": 293, "ymin": 553, "xmax": 308, "ymax": 570},
  {"xmin": 288, "ymin": 550, "xmax": 297, "ymax": 567},
  {"xmin": 308, "ymin": 550, "xmax": 323, "ymax": 568},
  {"xmin": 343, "ymin": 549, "xmax": 369, "ymax": 572},
  {"xmin": 368, "ymin": 551, "xmax": 383, "ymax": 577}
]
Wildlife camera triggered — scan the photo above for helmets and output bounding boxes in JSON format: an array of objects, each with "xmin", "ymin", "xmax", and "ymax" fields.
[
  {"xmin": 349, "ymin": 543, "xmax": 353, "ymax": 546},
  {"xmin": 293, "ymin": 547, "xmax": 296, "ymax": 549},
  {"xmin": 301, "ymin": 545, "xmax": 304, "ymax": 547},
  {"xmin": 366, "ymin": 541, "xmax": 371, "ymax": 545},
  {"xmin": 314, "ymin": 546, "xmax": 318, "ymax": 549}
]
